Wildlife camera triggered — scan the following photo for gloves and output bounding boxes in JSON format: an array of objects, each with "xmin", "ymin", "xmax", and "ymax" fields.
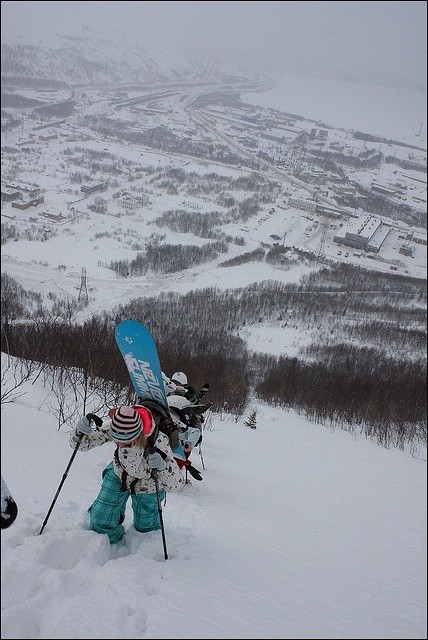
[
  {"xmin": 148, "ymin": 453, "xmax": 166, "ymax": 471},
  {"xmin": 76, "ymin": 417, "xmax": 93, "ymax": 436},
  {"xmin": 186, "ymin": 460, "xmax": 202, "ymax": 480}
]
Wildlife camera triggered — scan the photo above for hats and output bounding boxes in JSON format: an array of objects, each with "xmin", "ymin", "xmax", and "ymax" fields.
[{"xmin": 109, "ymin": 405, "xmax": 143, "ymax": 443}]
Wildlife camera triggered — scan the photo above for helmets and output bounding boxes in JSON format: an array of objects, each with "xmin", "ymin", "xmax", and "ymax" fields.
[{"xmin": 172, "ymin": 371, "xmax": 188, "ymax": 385}]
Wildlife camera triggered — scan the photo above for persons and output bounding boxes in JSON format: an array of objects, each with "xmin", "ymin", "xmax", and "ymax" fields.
[
  {"xmin": 169, "ymin": 370, "xmax": 203, "ymax": 448},
  {"xmin": 68, "ymin": 403, "xmax": 186, "ymax": 545},
  {"xmin": 164, "ymin": 407, "xmax": 202, "ymax": 486}
]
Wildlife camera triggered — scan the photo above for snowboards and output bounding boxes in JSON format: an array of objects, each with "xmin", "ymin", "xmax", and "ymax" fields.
[
  {"xmin": 196, "ymin": 383, "xmax": 209, "ymax": 401},
  {"xmin": 114, "ymin": 320, "xmax": 187, "ymax": 462}
]
